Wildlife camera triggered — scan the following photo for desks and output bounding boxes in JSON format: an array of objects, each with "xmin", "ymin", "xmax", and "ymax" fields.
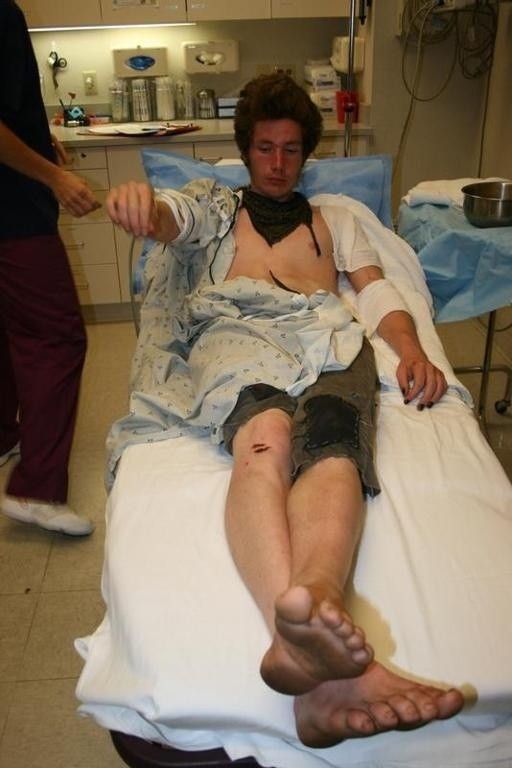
[{"xmin": 399, "ymin": 198, "xmax": 510, "ymax": 420}]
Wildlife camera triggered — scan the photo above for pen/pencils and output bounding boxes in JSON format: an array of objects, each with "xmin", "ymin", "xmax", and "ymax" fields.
[{"xmin": 142, "ymin": 127, "xmax": 166, "ymax": 131}]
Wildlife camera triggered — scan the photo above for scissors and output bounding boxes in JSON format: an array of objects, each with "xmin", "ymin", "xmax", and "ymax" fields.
[{"xmin": 48, "ymin": 52, "xmax": 66, "ymax": 89}]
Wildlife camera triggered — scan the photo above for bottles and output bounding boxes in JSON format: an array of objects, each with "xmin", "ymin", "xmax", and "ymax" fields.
[
  {"xmin": 109, "ymin": 88, "xmax": 130, "ymax": 122},
  {"xmin": 152, "ymin": 76, "xmax": 177, "ymax": 120},
  {"xmin": 173, "ymin": 77, "xmax": 196, "ymax": 119},
  {"xmin": 196, "ymin": 87, "xmax": 217, "ymax": 120},
  {"xmin": 130, "ymin": 79, "xmax": 150, "ymax": 123}
]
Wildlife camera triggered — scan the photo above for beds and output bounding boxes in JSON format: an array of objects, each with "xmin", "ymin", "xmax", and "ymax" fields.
[{"xmin": 94, "ymin": 160, "xmax": 508, "ymax": 765}]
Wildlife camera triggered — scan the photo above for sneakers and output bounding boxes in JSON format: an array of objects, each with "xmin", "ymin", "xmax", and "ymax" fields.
[
  {"xmin": 0, "ymin": 426, "xmax": 20, "ymax": 468},
  {"xmin": 1, "ymin": 495, "xmax": 95, "ymax": 537}
]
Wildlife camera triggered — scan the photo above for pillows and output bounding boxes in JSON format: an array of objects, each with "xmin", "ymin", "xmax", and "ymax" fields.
[{"xmin": 129, "ymin": 149, "xmax": 393, "ymax": 295}]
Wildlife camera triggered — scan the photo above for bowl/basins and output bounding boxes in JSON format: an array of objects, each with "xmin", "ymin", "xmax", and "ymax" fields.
[{"xmin": 460, "ymin": 180, "xmax": 512, "ymax": 229}]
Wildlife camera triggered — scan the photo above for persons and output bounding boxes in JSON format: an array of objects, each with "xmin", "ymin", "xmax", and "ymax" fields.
[
  {"xmin": 103, "ymin": 66, "xmax": 465, "ymax": 750},
  {"xmin": 1, "ymin": 0, "xmax": 105, "ymax": 538}
]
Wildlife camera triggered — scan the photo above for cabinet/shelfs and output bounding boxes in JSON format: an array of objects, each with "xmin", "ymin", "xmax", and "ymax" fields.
[
  {"xmin": 310, "ymin": 137, "xmax": 372, "ymax": 158},
  {"xmin": 196, "ymin": 142, "xmax": 241, "ymax": 158},
  {"xmin": 58, "ymin": 145, "xmax": 122, "ymax": 306},
  {"xmin": 106, "ymin": 141, "xmax": 194, "ymax": 302}
]
